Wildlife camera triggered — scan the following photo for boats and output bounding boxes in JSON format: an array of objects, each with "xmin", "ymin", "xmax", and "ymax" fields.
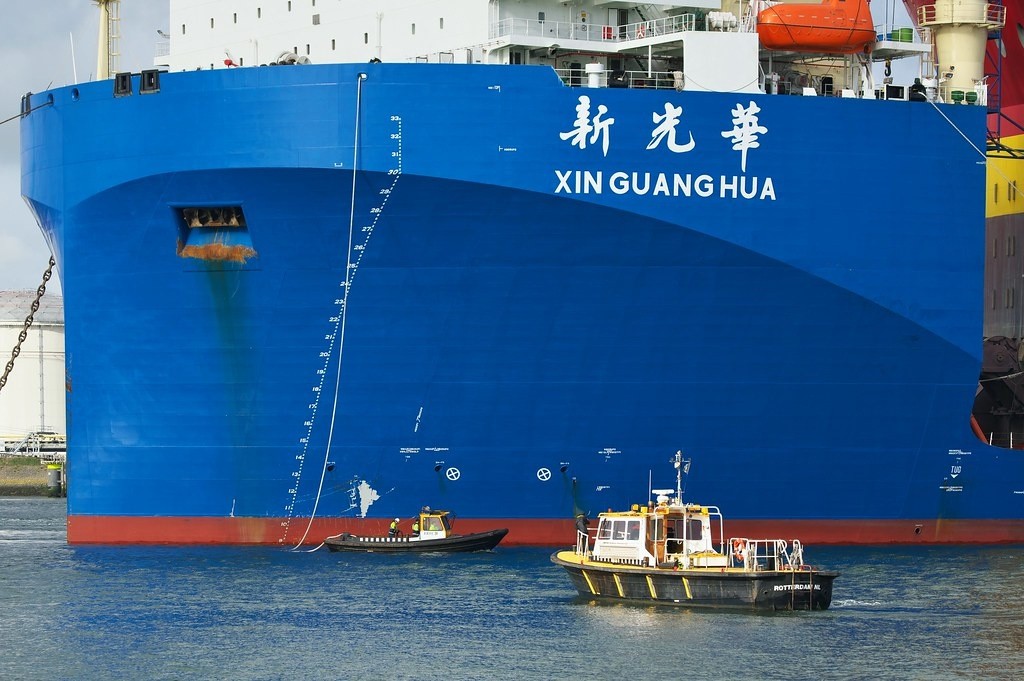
[
  {"xmin": 757, "ymin": 0, "xmax": 878, "ymax": 54},
  {"xmin": 324, "ymin": 498, "xmax": 512, "ymax": 554},
  {"xmin": 548, "ymin": 443, "xmax": 841, "ymax": 616}
]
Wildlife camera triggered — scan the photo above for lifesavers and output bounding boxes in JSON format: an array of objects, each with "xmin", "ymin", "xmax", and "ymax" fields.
[
  {"xmin": 733, "ymin": 539, "xmax": 745, "ymax": 560},
  {"xmin": 609, "ymin": 69, "xmax": 629, "ymax": 88},
  {"xmin": 637, "ymin": 25, "xmax": 646, "ymax": 39}
]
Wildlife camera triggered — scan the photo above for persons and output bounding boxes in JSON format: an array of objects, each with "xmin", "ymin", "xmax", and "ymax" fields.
[
  {"xmin": 389, "ymin": 517, "xmax": 400, "ymax": 538},
  {"xmin": 575, "ymin": 512, "xmax": 591, "ymax": 556},
  {"xmin": 412, "ymin": 520, "xmax": 420, "ymax": 537},
  {"xmin": 428, "ymin": 521, "xmax": 439, "ymax": 530}
]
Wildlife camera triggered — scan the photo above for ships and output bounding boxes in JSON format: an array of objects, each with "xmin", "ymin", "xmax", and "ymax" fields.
[{"xmin": 19, "ymin": 0, "xmax": 1024, "ymax": 550}]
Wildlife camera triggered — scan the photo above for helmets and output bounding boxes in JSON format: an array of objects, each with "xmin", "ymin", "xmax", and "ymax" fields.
[{"xmin": 395, "ymin": 518, "xmax": 400, "ymax": 523}]
